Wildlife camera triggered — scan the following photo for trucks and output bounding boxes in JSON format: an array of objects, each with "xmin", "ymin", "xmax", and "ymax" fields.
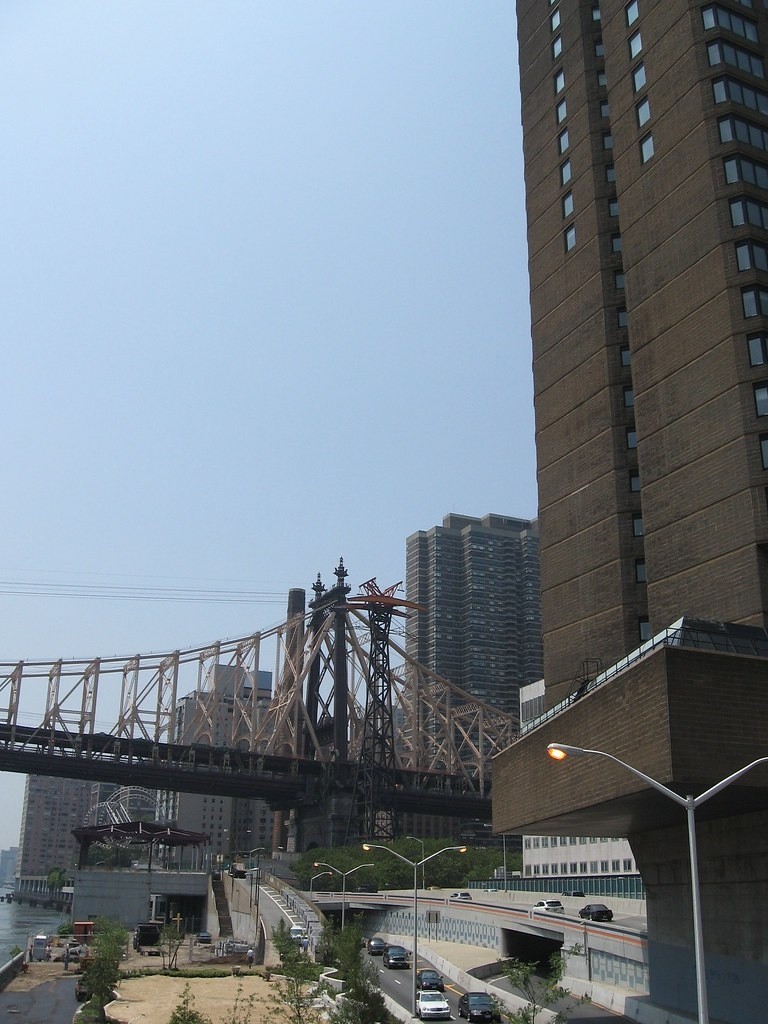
[{"xmin": 133, "ymin": 922, "xmax": 165, "ymax": 957}]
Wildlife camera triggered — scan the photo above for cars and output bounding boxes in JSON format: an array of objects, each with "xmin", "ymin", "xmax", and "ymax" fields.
[
  {"xmin": 427, "ymin": 887, "xmax": 445, "ymax": 892},
  {"xmin": 360, "ymin": 937, "xmax": 411, "ymax": 969},
  {"xmin": 416, "ymin": 968, "xmax": 444, "ymax": 992},
  {"xmin": 458, "ymin": 992, "xmax": 501, "ymax": 1024},
  {"xmin": 532, "ymin": 899, "xmax": 564, "ymax": 914},
  {"xmin": 416, "ymin": 990, "xmax": 451, "ymax": 1021},
  {"xmin": 198, "ymin": 932, "xmax": 211, "ymax": 944},
  {"xmin": 449, "ymin": 892, "xmax": 472, "ymax": 900},
  {"xmin": 560, "ymin": 891, "xmax": 585, "ymax": 897},
  {"xmin": 483, "ymin": 889, "xmax": 498, "ymax": 895},
  {"xmin": 578, "ymin": 904, "xmax": 614, "ymax": 921}
]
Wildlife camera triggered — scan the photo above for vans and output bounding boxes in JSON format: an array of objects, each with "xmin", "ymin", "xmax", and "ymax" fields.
[
  {"xmin": 228, "ymin": 862, "xmax": 246, "ymax": 879},
  {"xmin": 290, "ymin": 926, "xmax": 303, "ymax": 947}
]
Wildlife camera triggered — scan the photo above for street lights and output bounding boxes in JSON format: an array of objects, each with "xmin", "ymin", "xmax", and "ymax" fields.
[
  {"xmin": 362, "ymin": 843, "xmax": 466, "ymax": 1021},
  {"xmin": 547, "ymin": 743, "xmax": 768, "ymax": 1024},
  {"xmin": 484, "ymin": 824, "xmax": 507, "ymax": 893},
  {"xmin": 243, "ymin": 846, "xmax": 265, "ymax": 943},
  {"xmin": 407, "ymin": 837, "xmax": 424, "ymax": 890},
  {"xmin": 310, "ymin": 872, "xmax": 332, "ymax": 900},
  {"xmin": 273, "ymin": 844, "xmax": 283, "ymax": 876},
  {"xmin": 314, "ymin": 862, "xmax": 375, "ymax": 933}
]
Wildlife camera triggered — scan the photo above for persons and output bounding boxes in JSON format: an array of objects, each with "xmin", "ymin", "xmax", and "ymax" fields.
[
  {"xmin": 329, "ymin": 743, "xmax": 336, "ymax": 764},
  {"xmin": 302, "ymin": 938, "xmax": 310, "ymax": 956},
  {"xmin": 247, "ymin": 948, "xmax": 253, "ymax": 969}
]
